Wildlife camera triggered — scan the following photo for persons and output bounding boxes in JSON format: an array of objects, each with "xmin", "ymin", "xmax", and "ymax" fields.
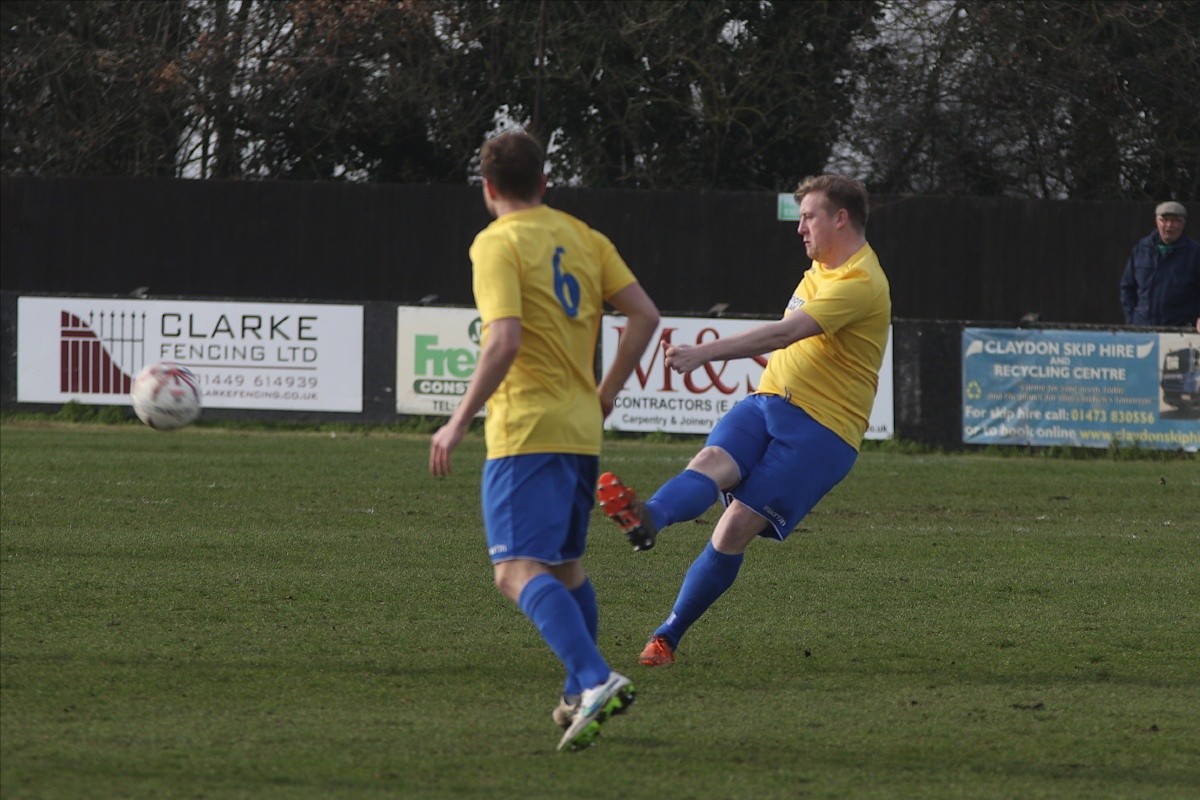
[
  {"xmin": 429, "ymin": 127, "xmax": 660, "ymax": 752},
  {"xmin": 600, "ymin": 172, "xmax": 893, "ymax": 668},
  {"xmin": 1120, "ymin": 200, "xmax": 1200, "ymax": 334}
]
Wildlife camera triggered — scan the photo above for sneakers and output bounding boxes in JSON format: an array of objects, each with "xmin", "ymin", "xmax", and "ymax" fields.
[
  {"xmin": 557, "ymin": 670, "xmax": 635, "ymax": 753},
  {"xmin": 553, "ymin": 695, "xmax": 578, "ymax": 729},
  {"xmin": 638, "ymin": 636, "xmax": 675, "ymax": 666},
  {"xmin": 598, "ymin": 471, "xmax": 655, "ymax": 552}
]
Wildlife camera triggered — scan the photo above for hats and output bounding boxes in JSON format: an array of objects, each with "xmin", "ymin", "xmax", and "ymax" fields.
[{"xmin": 1155, "ymin": 202, "xmax": 1186, "ymax": 217}]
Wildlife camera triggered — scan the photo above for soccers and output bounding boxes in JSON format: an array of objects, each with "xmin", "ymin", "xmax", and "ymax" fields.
[{"xmin": 131, "ymin": 362, "xmax": 205, "ymax": 431}]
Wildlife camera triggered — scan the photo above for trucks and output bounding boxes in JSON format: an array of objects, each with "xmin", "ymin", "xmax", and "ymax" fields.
[{"xmin": 1162, "ymin": 348, "xmax": 1200, "ymax": 416}]
